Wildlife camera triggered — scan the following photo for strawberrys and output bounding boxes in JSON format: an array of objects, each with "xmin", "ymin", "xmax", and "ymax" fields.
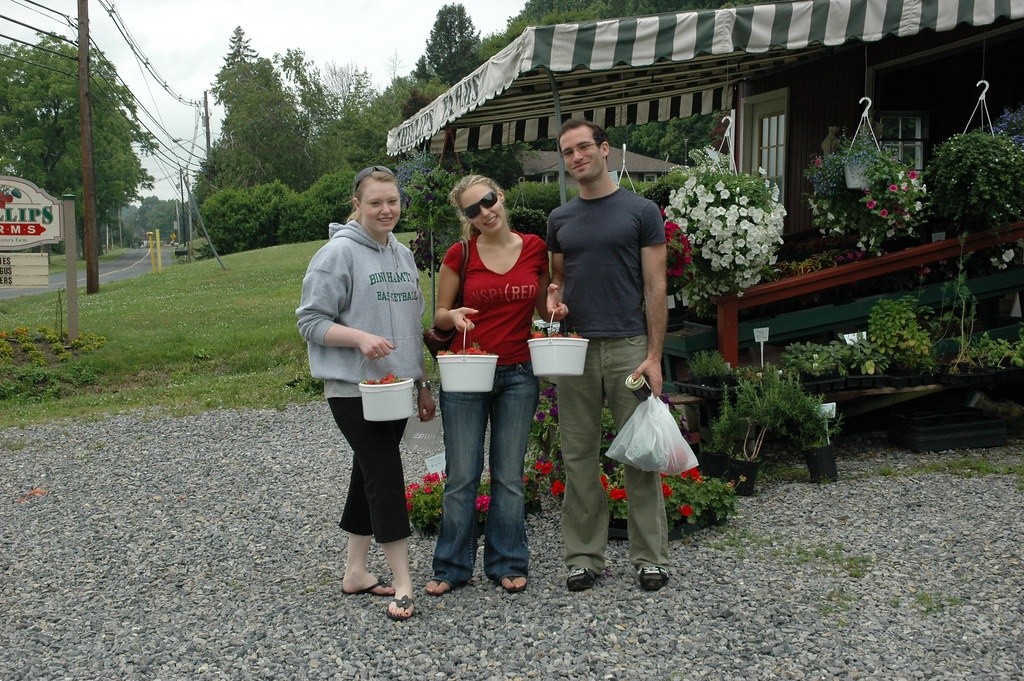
[
  {"xmin": 364, "ymin": 373, "xmax": 395, "ymax": 384},
  {"xmin": 437, "ymin": 342, "xmax": 490, "ymax": 354},
  {"xmin": 532, "ymin": 328, "xmax": 582, "ymax": 338}
]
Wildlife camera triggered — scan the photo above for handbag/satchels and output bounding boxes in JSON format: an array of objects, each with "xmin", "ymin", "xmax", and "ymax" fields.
[
  {"xmin": 603, "ymin": 389, "xmax": 699, "ymax": 476},
  {"xmin": 422, "ymin": 239, "xmax": 470, "ymax": 362}
]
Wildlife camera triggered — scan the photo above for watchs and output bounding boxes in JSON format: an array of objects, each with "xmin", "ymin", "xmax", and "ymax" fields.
[{"xmin": 417, "ymin": 381, "xmax": 431, "ymax": 390}]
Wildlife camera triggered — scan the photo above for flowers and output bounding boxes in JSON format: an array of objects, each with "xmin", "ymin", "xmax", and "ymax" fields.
[
  {"xmin": 504, "ymin": 205, "xmax": 548, "ymax": 241},
  {"xmin": 660, "ymin": 144, "xmax": 788, "ymax": 319},
  {"xmin": 409, "ymin": 230, "xmax": 464, "ymax": 278},
  {"xmin": 797, "ymin": 124, "xmax": 935, "ymax": 258},
  {"xmin": 924, "ymin": 126, "xmax": 1024, "ymax": 239},
  {"xmin": 535, "ymin": 459, "xmax": 747, "ymax": 532},
  {"xmin": 401, "ymin": 166, "xmax": 465, "ymax": 230},
  {"xmin": 991, "ymin": 107, "xmax": 1024, "ymax": 153},
  {"xmin": 520, "ymin": 383, "xmax": 693, "ymax": 472},
  {"xmin": 402, "ymin": 471, "xmax": 546, "ymax": 535}
]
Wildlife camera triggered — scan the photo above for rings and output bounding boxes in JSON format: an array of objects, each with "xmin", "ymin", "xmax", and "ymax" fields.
[{"xmin": 373, "ymin": 354, "xmax": 378, "ymax": 358}]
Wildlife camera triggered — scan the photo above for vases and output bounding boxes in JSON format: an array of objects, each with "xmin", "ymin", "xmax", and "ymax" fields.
[
  {"xmin": 885, "ymin": 409, "xmax": 1010, "ymax": 454},
  {"xmin": 607, "ymin": 509, "xmax": 717, "ymax": 541},
  {"xmin": 542, "ymin": 426, "xmax": 616, "ymax": 454},
  {"xmin": 841, "ymin": 164, "xmax": 869, "ymax": 190},
  {"xmin": 422, "ymin": 498, "xmax": 542, "ymax": 536}
]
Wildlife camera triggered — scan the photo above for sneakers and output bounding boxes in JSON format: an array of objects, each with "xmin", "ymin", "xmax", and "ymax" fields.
[
  {"xmin": 566, "ymin": 567, "xmax": 595, "ymax": 592},
  {"xmin": 637, "ymin": 566, "xmax": 669, "ymax": 592}
]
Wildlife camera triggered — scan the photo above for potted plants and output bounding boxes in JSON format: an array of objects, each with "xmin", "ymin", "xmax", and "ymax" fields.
[{"xmin": 676, "ymin": 231, "xmax": 1024, "ymax": 497}]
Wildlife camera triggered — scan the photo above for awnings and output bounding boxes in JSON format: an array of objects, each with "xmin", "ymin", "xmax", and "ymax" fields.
[{"xmin": 385, "ymin": 0, "xmax": 1024, "ymax": 156}]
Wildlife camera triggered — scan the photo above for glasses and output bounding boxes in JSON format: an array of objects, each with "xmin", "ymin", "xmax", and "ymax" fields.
[
  {"xmin": 560, "ymin": 142, "xmax": 599, "ymax": 160},
  {"xmin": 355, "ymin": 166, "xmax": 395, "ymax": 194},
  {"xmin": 462, "ymin": 190, "xmax": 498, "ymax": 219}
]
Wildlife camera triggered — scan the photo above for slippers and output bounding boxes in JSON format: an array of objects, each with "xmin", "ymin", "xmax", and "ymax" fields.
[
  {"xmin": 341, "ymin": 574, "xmax": 395, "ymax": 597},
  {"xmin": 386, "ymin": 595, "xmax": 414, "ymax": 620}
]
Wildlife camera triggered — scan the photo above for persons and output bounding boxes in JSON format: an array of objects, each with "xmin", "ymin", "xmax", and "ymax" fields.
[
  {"xmin": 295, "ymin": 166, "xmax": 437, "ymax": 622},
  {"xmin": 426, "ymin": 176, "xmax": 559, "ymax": 595},
  {"xmin": 545, "ymin": 120, "xmax": 669, "ymax": 591}
]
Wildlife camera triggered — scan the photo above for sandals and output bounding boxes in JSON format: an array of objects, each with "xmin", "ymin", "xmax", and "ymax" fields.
[
  {"xmin": 500, "ymin": 575, "xmax": 527, "ymax": 593},
  {"xmin": 425, "ymin": 579, "xmax": 452, "ymax": 596}
]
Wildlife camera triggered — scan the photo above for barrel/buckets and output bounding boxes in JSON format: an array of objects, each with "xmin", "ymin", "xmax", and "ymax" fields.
[
  {"xmin": 435, "ymin": 354, "xmax": 499, "ymax": 393},
  {"xmin": 359, "ymin": 377, "xmax": 413, "ymax": 422},
  {"xmin": 525, "ymin": 338, "xmax": 590, "ymax": 377}
]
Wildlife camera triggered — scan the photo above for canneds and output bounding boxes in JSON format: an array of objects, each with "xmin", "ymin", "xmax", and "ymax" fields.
[{"xmin": 625, "ymin": 372, "xmax": 651, "ymax": 401}]
